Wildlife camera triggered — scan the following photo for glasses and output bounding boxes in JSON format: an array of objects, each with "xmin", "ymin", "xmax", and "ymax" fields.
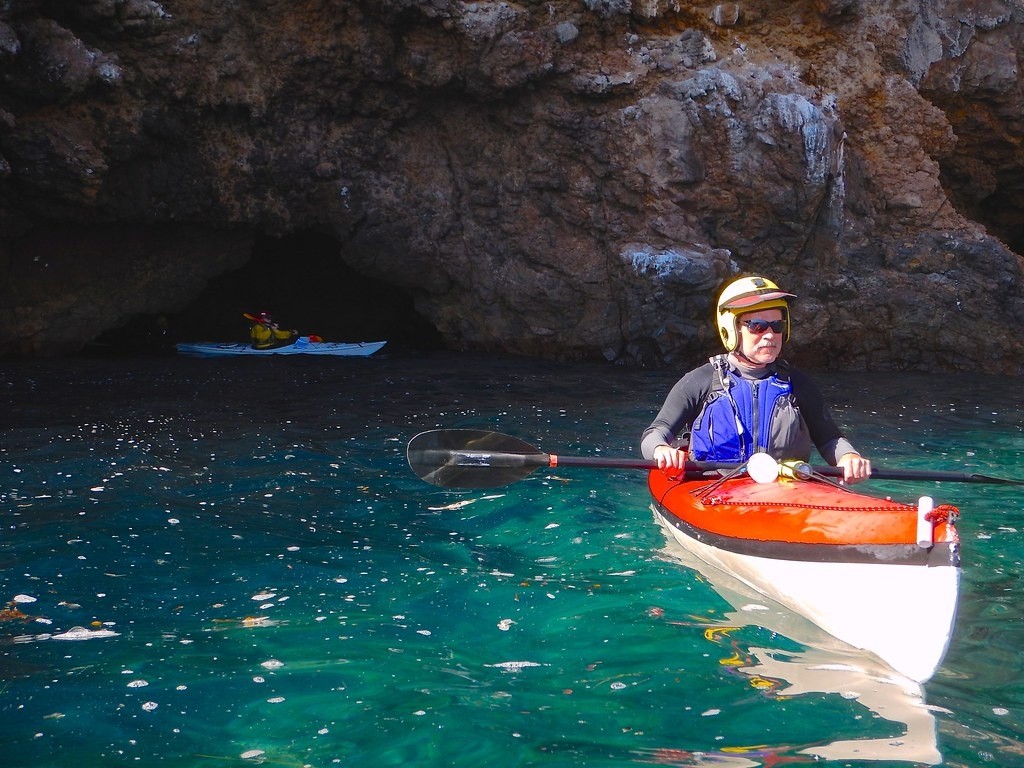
[{"xmin": 740, "ymin": 319, "xmax": 786, "ymax": 335}]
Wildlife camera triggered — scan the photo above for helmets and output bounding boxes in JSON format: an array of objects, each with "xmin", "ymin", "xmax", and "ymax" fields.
[
  {"xmin": 258, "ymin": 312, "xmax": 272, "ymax": 318},
  {"xmin": 717, "ymin": 276, "xmax": 797, "ymax": 351}
]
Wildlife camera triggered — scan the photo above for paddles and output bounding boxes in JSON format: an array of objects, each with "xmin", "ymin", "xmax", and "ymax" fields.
[
  {"xmin": 243, "ymin": 313, "xmax": 323, "ymax": 342},
  {"xmin": 406, "ymin": 425, "xmax": 1024, "ymax": 492}
]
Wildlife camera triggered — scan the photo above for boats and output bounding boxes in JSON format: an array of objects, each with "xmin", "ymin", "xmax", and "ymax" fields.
[
  {"xmin": 175, "ymin": 336, "xmax": 387, "ymax": 356},
  {"xmin": 645, "ymin": 426, "xmax": 963, "ymax": 685}
]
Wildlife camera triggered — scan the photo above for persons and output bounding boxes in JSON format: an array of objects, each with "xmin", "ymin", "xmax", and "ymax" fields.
[
  {"xmin": 251, "ymin": 312, "xmax": 297, "ymax": 349},
  {"xmin": 640, "ymin": 277, "xmax": 872, "ymax": 487}
]
